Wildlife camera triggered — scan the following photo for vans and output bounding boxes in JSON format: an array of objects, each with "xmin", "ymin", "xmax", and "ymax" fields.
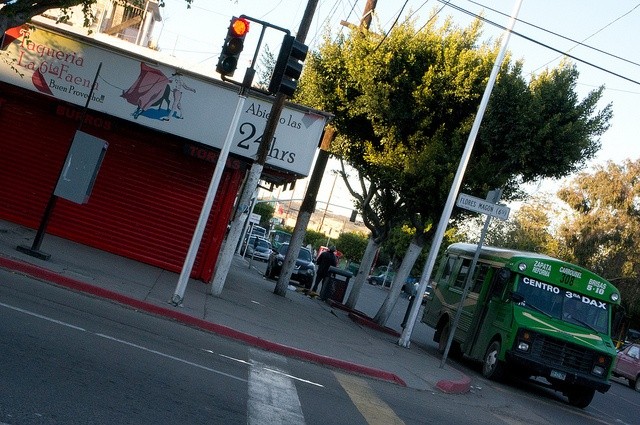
[{"xmin": 614, "ymin": 339, "xmax": 640, "ymax": 394}]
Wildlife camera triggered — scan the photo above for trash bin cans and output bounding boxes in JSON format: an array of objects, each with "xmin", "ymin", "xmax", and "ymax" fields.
[{"xmin": 319, "ymin": 266, "xmax": 354, "ymax": 304}]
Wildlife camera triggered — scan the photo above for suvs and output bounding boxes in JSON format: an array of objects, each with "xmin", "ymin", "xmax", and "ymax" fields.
[{"xmin": 266, "ymin": 242, "xmax": 315, "ymax": 291}]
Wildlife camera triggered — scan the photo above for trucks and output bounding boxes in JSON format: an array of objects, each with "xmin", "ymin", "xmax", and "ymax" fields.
[
  {"xmin": 247, "ymin": 225, "xmax": 268, "ymax": 244},
  {"xmin": 314, "ymin": 245, "xmax": 344, "ymax": 270}
]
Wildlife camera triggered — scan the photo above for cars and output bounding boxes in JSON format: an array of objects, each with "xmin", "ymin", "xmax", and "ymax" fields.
[
  {"xmin": 404, "ymin": 281, "xmax": 432, "ymax": 305},
  {"xmin": 242, "ymin": 235, "xmax": 269, "ymax": 263},
  {"xmin": 367, "ymin": 272, "xmax": 406, "ymax": 291}
]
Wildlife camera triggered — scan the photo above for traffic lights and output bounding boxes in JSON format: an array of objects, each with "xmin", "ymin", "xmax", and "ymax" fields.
[
  {"xmin": 267, "ymin": 33, "xmax": 311, "ymax": 100},
  {"xmin": 216, "ymin": 17, "xmax": 252, "ymax": 78},
  {"xmin": 348, "ymin": 209, "xmax": 357, "ymax": 223}
]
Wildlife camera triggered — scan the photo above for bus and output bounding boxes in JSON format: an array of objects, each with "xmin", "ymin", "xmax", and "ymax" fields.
[
  {"xmin": 268, "ymin": 229, "xmax": 298, "ymax": 257},
  {"xmin": 420, "ymin": 239, "xmax": 623, "ymax": 408}
]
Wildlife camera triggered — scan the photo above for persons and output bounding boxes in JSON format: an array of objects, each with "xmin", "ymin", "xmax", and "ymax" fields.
[
  {"xmin": 313, "ymin": 246, "xmax": 337, "ymax": 293},
  {"xmin": 160, "ymin": 72, "xmax": 196, "ymax": 121}
]
